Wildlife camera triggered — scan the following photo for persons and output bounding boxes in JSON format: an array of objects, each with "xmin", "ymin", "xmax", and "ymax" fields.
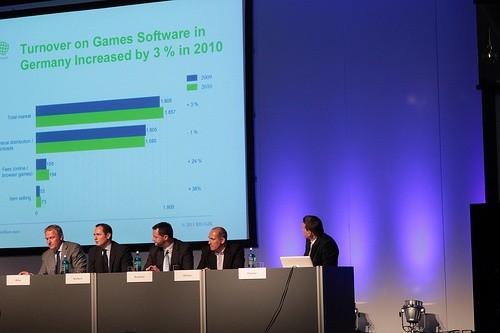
[
  {"xmin": 141, "ymin": 221, "xmax": 194, "ymax": 272},
  {"xmin": 16, "ymin": 224, "xmax": 87, "ymax": 275},
  {"xmin": 197, "ymin": 227, "xmax": 245, "ymax": 271},
  {"xmin": 301, "ymin": 215, "xmax": 339, "ymax": 267},
  {"xmin": 87, "ymin": 224, "xmax": 135, "ymax": 272}
]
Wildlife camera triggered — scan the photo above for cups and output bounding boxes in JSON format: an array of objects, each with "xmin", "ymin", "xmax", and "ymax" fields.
[
  {"xmin": 258, "ymin": 262, "xmax": 264, "ymax": 268},
  {"xmin": 173, "ymin": 265, "xmax": 179, "ymax": 270}
]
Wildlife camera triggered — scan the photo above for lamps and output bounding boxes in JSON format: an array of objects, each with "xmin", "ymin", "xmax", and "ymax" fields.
[{"xmin": 399, "ymin": 300, "xmax": 425, "ymax": 333}]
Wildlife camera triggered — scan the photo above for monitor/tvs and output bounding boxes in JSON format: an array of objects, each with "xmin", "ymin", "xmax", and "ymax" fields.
[{"xmin": 279, "ymin": 255, "xmax": 314, "ymax": 268}]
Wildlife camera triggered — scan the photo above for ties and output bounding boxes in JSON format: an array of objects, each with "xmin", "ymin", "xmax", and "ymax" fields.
[
  {"xmin": 56, "ymin": 251, "xmax": 60, "ymax": 274},
  {"xmin": 163, "ymin": 250, "xmax": 169, "ymax": 272},
  {"xmin": 102, "ymin": 249, "xmax": 108, "ymax": 273}
]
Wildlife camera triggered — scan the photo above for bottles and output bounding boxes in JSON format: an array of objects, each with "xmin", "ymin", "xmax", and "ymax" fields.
[
  {"xmin": 247, "ymin": 248, "xmax": 256, "ymax": 268},
  {"xmin": 61, "ymin": 254, "xmax": 69, "ymax": 274},
  {"xmin": 134, "ymin": 251, "xmax": 142, "ymax": 271}
]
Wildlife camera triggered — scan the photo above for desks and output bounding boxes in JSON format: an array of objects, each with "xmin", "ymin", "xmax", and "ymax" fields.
[{"xmin": 0, "ymin": 266, "xmax": 356, "ymax": 333}]
